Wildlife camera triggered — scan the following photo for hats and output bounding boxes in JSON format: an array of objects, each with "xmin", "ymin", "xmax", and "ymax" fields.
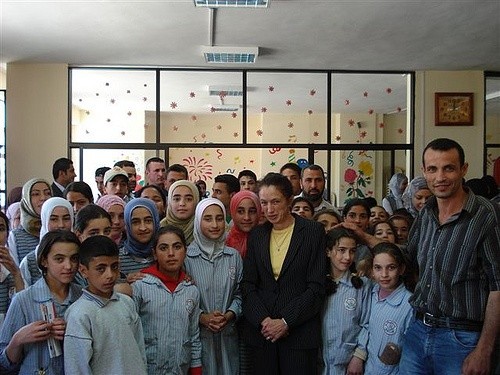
[{"xmin": 103, "ymin": 166, "xmax": 129, "ymax": 186}]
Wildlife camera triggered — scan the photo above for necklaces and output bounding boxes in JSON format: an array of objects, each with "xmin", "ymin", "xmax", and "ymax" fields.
[{"xmin": 273, "ymin": 228, "xmax": 291, "ymax": 252}]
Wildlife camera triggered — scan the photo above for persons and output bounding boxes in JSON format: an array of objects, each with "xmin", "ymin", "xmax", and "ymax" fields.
[
  {"xmin": 319, "ymin": 226, "xmax": 371, "ymax": 375},
  {"xmin": 0, "ymin": 229, "xmax": 84, "ymax": 375},
  {"xmin": 182, "ymin": 198, "xmax": 243, "ymax": 375},
  {"xmin": 0, "ymin": 157, "xmax": 433, "ymax": 325},
  {"xmin": 463, "ymin": 175, "xmax": 500, "ymax": 212},
  {"xmin": 240, "ymin": 173, "xmax": 330, "ymax": 375},
  {"xmin": 363, "ymin": 242, "xmax": 414, "ymax": 375},
  {"xmin": 130, "ymin": 225, "xmax": 202, "ymax": 375},
  {"xmin": 63, "ymin": 236, "xmax": 147, "ymax": 375},
  {"xmin": 328, "ymin": 138, "xmax": 500, "ymax": 375}
]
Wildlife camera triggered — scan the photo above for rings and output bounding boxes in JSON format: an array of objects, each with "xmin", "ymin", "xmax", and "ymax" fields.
[{"xmin": 267, "ymin": 336, "xmax": 271, "ymax": 340}]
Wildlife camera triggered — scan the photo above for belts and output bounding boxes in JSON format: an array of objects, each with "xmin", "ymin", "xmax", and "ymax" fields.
[{"xmin": 414, "ymin": 311, "xmax": 482, "ymax": 331}]
